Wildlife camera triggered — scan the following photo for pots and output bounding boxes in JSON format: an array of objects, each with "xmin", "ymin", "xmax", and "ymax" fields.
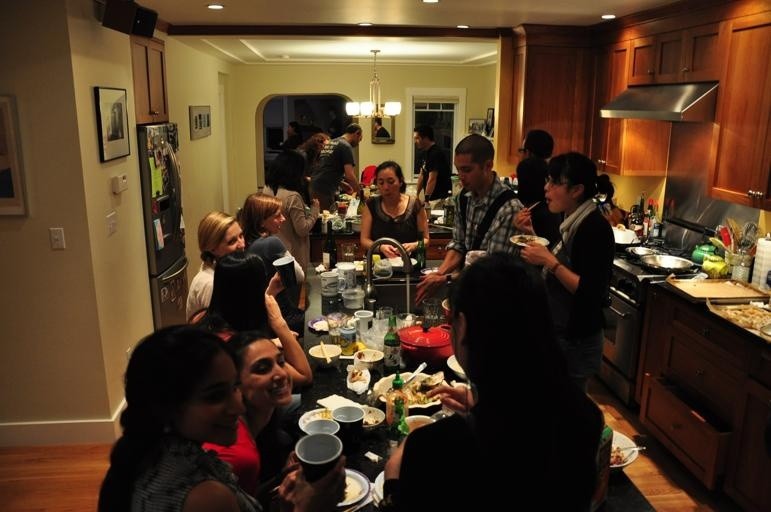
[{"xmin": 611, "ymin": 224, "xmax": 694, "ymax": 273}]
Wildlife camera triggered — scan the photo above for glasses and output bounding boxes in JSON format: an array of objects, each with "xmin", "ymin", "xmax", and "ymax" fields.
[{"xmin": 544, "ymin": 176, "xmax": 568, "ymax": 187}]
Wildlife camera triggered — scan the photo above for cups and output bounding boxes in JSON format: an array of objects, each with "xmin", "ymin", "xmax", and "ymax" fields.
[{"xmin": 295, "ymin": 242, "xmax": 450, "ymax": 482}]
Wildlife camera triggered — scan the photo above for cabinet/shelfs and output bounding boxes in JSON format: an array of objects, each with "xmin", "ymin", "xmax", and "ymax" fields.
[
  {"xmin": 633, "ymin": 280, "xmax": 770, "ymax": 512},
  {"xmin": 130, "ymin": 34, "xmax": 170, "ymax": 127}
]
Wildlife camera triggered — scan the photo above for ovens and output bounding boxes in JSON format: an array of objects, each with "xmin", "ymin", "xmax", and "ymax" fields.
[{"xmin": 597, "ymin": 284, "xmax": 639, "ymax": 406}]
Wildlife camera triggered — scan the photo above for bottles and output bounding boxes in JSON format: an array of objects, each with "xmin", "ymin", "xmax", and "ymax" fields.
[
  {"xmin": 628, "ymin": 192, "xmax": 663, "ymax": 241},
  {"xmin": 321, "ymin": 184, "xmax": 455, "ymax": 458}
]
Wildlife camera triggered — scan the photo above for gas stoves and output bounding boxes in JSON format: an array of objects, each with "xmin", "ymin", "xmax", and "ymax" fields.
[{"xmin": 609, "ymin": 250, "xmax": 702, "ymax": 308}]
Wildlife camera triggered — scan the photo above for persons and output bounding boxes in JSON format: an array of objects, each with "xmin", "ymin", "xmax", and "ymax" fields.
[
  {"xmin": 413, "ymin": 125, "xmax": 454, "ymax": 206},
  {"xmin": 358, "ymin": 159, "xmax": 431, "ymax": 261},
  {"xmin": 381, "ymin": 248, "xmax": 605, "ymax": 511},
  {"xmin": 513, "ymin": 151, "xmax": 616, "ymax": 388},
  {"xmin": 516, "ymin": 128, "xmax": 565, "ymax": 246},
  {"xmin": 94, "ymin": 122, "xmax": 365, "ymax": 512},
  {"xmin": 375, "ymin": 118, "xmax": 391, "ymax": 138},
  {"xmin": 413, "ymin": 131, "xmax": 529, "ymax": 306}
]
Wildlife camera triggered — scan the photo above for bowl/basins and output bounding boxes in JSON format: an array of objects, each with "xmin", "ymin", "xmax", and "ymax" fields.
[{"xmin": 609, "ymin": 431, "xmax": 638, "ymax": 468}]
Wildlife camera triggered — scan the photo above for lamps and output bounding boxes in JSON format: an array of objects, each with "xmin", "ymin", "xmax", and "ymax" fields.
[{"xmin": 343, "ymin": 48, "xmax": 403, "ymax": 118}]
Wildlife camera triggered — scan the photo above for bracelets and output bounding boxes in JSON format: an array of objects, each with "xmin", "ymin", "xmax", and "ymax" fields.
[{"xmin": 548, "ymin": 262, "xmax": 561, "ymax": 278}]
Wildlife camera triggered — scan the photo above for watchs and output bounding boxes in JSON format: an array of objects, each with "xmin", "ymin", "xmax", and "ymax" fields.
[{"xmin": 445, "ymin": 274, "xmax": 453, "ymax": 288}]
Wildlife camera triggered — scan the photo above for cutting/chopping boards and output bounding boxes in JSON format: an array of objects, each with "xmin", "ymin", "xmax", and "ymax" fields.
[{"xmin": 665, "ymin": 278, "xmax": 770, "ymax": 304}]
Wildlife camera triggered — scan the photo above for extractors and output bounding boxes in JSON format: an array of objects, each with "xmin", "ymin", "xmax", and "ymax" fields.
[{"xmin": 599, "ymin": 79, "xmax": 719, "ymax": 122}]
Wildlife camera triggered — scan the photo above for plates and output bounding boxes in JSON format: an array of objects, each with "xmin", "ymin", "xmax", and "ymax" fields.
[
  {"xmin": 510, "ymin": 235, "xmax": 549, "ymax": 247},
  {"xmin": 334, "ymin": 468, "xmax": 370, "ymax": 507}
]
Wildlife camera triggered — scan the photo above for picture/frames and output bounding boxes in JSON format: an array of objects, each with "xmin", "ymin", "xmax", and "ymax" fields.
[
  {"xmin": 467, "ymin": 118, "xmax": 486, "ymax": 135},
  {"xmin": 370, "ymin": 104, "xmax": 396, "ymax": 145},
  {"xmin": 187, "ymin": 104, "xmax": 213, "ymax": 141},
  {"xmin": 93, "ymin": 85, "xmax": 132, "ymax": 165},
  {"xmin": 485, "ymin": 107, "xmax": 494, "ymax": 127},
  {"xmin": 0, "ymin": 93, "xmax": 30, "ymax": 220}
]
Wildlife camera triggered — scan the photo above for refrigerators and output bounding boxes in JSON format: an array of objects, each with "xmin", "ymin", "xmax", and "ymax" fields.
[{"xmin": 137, "ymin": 122, "xmax": 189, "ymax": 332}]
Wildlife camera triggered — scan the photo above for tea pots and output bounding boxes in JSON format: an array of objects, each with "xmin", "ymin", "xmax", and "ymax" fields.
[
  {"xmin": 703, "ymin": 254, "xmax": 728, "ymax": 277},
  {"xmin": 692, "ymin": 244, "xmax": 717, "ymax": 264}
]
